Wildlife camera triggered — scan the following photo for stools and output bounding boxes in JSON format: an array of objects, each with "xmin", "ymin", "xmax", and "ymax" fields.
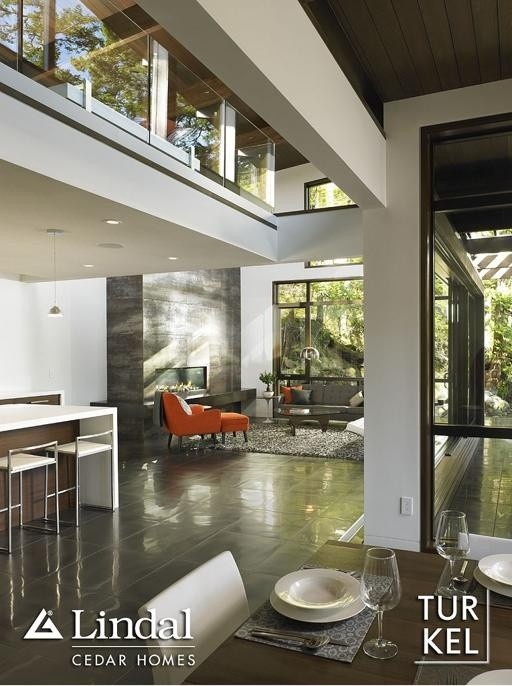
[{"xmin": 221, "ymin": 413, "xmax": 249, "ymax": 444}]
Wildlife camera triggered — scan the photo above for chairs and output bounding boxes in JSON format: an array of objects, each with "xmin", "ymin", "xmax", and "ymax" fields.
[
  {"xmin": 458, "ymin": 532, "xmax": 512, "ymax": 560},
  {"xmin": 139, "ymin": 550, "xmax": 252, "ymax": 685},
  {"xmin": 42, "ymin": 430, "xmax": 113, "ymax": 528},
  {"xmin": 0, "ymin": 441, "xmax": 59, "ymax": 554},
  {"xmin": 155, "ymin": 390, "xmax": 221, "ymax": 451}
]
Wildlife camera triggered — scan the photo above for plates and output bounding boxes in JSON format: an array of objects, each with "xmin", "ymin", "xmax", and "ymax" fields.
[
  {"xmin": 269, "ymin": 569, "xmax": 369, "ymax": 623},
  {"xmin": 473, "ymin": 554, "xmax": 512, "ymax": 597}
]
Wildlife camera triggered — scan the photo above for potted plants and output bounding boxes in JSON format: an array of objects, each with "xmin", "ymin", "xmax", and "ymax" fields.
[{"xmin": 258, "ymin": 370, "xmax": 278, "ymax": 398}]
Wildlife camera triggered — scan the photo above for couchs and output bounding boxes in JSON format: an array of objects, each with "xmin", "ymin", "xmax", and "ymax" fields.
[{"xmin": 275, "ymin": 384, "xmax": 364, "ymax": 425}]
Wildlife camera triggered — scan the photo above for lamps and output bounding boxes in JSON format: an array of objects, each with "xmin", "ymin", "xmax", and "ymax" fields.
[
  {"xmin": 45, "ymin": 230, "xmax": 63, "ymax": 317},
  {"xmin": 285, "ymin": 347, "xmax": 319, "ymax": 386}
]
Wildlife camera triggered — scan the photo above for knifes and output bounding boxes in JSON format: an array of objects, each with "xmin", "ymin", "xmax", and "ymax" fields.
[{"xmin": 253, "ymin": 627, "xmax": 350, "ymax": 647}]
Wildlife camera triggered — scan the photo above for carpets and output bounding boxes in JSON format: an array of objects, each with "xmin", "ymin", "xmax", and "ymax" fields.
[{"xmin": 173, "ymin": 423, "xmax": 364, "ymax": 462}]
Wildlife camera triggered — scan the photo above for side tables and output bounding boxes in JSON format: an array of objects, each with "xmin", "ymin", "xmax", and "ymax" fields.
[{"xmin": 255, "ymin": 396, "xmax": 281, "ymax": 423}]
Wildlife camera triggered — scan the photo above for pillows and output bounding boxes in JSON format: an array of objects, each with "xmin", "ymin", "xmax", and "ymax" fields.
[
  {"xmin": 290, "ymin": 387, "xmax": 313, "ymax": 404},
  {"xmin": 349, "ymin": 390, "xmax": 364, "ymax": 407},
  {"xmin": 281, "ymin": 385, "xmax": 302, "ymax": 404}
]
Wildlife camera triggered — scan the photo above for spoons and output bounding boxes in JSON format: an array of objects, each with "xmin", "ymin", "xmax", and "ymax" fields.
[
  {"xmin": 246, "ymin": 632, "xmax": 327, "ymax": 653},
  {"xmin": 452, "ymin": 559, "xmax": 470, "ymax": 583}
]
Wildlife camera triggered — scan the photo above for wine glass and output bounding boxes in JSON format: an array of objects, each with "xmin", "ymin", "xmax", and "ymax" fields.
[
  {"xmin": 435, "ymin": 510, "xmax": 475, "ymax": 607},
  {"xmin": 362, "ymin": 548, "xmax": 401, "ymax": 659}
]
